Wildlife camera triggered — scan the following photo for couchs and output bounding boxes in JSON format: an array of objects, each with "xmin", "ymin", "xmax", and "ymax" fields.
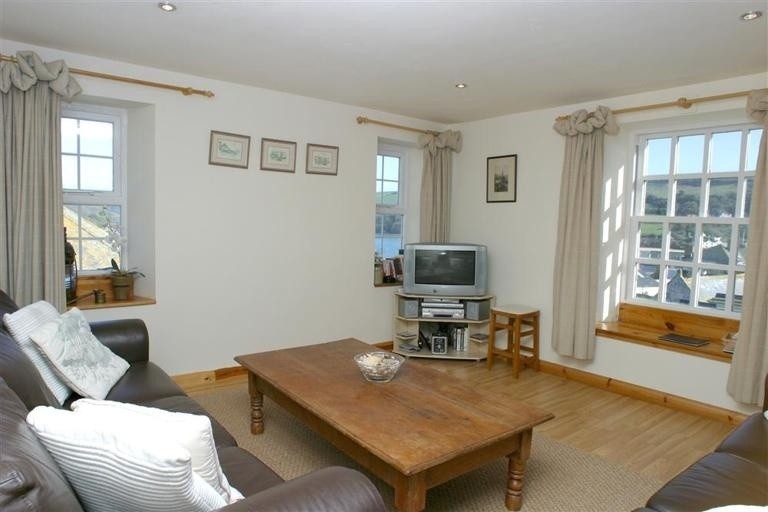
[
  {"xmin": 0, "ymin": 289, "xmax": 387, "ymax": 511},
  {"xmin": 634, "ymin": 375, "xmax": 768, "ymax": 512}
]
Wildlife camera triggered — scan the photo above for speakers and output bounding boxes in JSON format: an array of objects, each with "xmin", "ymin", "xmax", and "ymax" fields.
[
  {"xmin": 398, "ymin": 298, "xmax": 418, "ymax": 318},
  {"xmin": 466, "ymin": 300, "xmax": 490, "ymax": 321}
]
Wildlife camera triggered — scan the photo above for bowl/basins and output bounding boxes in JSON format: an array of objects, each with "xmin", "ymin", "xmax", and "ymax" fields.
[{"xmin": 353, "ymin": 351, "xmax": 406, "ymax": 386}]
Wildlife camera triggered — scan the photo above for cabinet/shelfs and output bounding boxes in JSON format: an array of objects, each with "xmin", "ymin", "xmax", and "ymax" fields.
[{"xmin": 392, "ymin": 292, "xmax": 493, "ymax": 362}]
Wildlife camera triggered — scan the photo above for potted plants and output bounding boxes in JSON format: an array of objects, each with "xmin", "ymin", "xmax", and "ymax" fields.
[{"xmin": 99, "ymin": 260, "xmax": 146, "ymax": 300}]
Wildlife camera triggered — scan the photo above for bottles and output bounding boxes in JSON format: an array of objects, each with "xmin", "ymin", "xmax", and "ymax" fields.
[{"xmin": 395, "ymin": 248, "xmax": 405, "ymax": 282}]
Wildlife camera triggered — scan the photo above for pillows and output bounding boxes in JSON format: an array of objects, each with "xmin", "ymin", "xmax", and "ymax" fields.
[
  {"xmin": 2, "ymin": 298, "xmax": 71, "ymax": 406},
  {"xmin": 25, "ymin": 407, "xmax": 226, "ymax": 511},
  {"xmin": 71, "ymin": 399, "xmax": 245, "ymax": 503},
  {"xmin": 30, "ymin": 308, "xmax": 130, "ymax": 399}
]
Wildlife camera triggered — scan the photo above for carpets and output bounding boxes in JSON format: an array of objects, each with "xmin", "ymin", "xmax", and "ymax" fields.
[{"xmin": 189, "ymin": 386, "xmax": 663, "ymax": 511}]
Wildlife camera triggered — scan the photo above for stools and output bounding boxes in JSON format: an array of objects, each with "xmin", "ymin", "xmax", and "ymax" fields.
[{"xmin": 488, "ymin": 306, "xmax": 538, "ymax": 379}]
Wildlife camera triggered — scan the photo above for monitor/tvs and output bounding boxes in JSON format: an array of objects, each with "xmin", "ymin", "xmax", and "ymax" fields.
[{"xmin": 404, "ymin": 242, "xmax": 486, "ymax": 296}]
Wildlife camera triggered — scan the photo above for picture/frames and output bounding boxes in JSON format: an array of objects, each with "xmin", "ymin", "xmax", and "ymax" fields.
[
  {"xmin": 486, "ymin": 154, "xmax": 516, "ymax": 202},
  {"xmin": 209, "ymin": 131, "xmax": 251, "ymax": 169},
  {"xmin": 260, "ymin": 139, "xmax": 297, "ymax": 173},
  {"xmin": 306, "ymin": 144, "xmax": 339, "ymax": 176}
]
